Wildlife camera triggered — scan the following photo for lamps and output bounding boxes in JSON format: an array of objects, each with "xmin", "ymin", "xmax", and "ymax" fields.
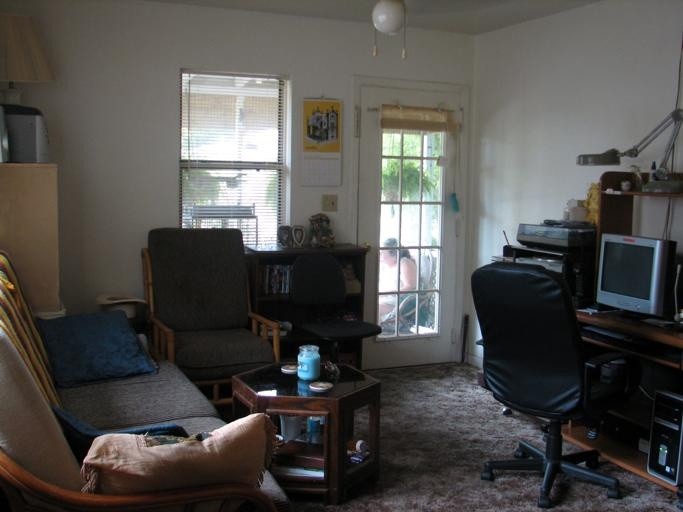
[
  {"xmin": 371, "ymin": 0, "xmax": 408, "ymax": 59},
  {"xmin": 576, "ymin": 109, "xmax": 683, "ymax": 192},
  {"xmin": 0, "ymin": 12, "xmax": 56, "ymax": 105}
]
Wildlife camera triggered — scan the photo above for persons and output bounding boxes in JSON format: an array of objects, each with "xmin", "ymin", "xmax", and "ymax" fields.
[
  {"xmin": 380, "ymin": 237, "xmax": 426, "ymax": 334},
  {"xmin": 342, "ymin": 264, "xmax": 360, "ymax": 294}
]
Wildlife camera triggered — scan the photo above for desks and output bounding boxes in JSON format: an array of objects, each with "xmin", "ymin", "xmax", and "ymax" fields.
[{"xmin": 568, "ymin": 308, "xmax": 683, "ymax": 493}]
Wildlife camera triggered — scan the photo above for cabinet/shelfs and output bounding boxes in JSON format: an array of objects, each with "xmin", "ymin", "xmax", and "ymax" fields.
[
  {"xmin": 0, "ymin": 164, "xmax": 63, "ymax": 317},
  {"xmin": 597, "ymin": 169, "xmax": 683, "ymax": 312},
  {"xmin": 282, "ymin": 242, "xmax": 382, "ymax": 372},
  {"xmin": 501, "ymin": 245, "xmax": 595, "ymax": 302}
]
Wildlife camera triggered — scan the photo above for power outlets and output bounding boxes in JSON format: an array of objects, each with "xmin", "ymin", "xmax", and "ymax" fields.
[{"xmin": 321, "ymin": 194, "xmax": 338, "ymax": 212}]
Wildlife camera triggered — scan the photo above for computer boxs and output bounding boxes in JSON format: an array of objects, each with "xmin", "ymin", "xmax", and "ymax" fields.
[{"xmin": 646, "ymin": 387, "xmax": 683, "ymax": 487}]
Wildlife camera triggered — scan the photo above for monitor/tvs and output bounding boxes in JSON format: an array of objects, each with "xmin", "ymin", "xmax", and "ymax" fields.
[{"xmin": 595, "ymin": 232, "xmax": 678, "ymax": 316}]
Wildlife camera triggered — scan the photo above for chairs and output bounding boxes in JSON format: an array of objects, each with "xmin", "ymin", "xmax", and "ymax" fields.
[
  {"xmin": 473, "ymin": 263, "xmax": 631, "ymax": 507},
  {"xmin": 380, "ymin": 248, "xmax": 437, "ymax": 336},
  {"xmin": 142, "ymin": 227, "xmax": 281, "ymax": 407}
]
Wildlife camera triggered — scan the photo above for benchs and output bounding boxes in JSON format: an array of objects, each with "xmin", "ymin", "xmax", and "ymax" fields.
[{"xmin": 0, "ymin": 250, "xmax": 289, "ymax": 511}]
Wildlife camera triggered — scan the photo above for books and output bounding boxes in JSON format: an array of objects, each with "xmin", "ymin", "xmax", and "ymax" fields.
[
  {"xmin": 271, "ymin": 441, "xmax": 325, "ymax": 481},
  {"xmin": 264, "ymin": 265, "xmax": 292, "ymax": 295}
]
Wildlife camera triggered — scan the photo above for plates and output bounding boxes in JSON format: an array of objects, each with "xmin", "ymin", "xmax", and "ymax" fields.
[{"xmin": 308, "ymin": 381, "xmax": 334, "ymax": 393}]
